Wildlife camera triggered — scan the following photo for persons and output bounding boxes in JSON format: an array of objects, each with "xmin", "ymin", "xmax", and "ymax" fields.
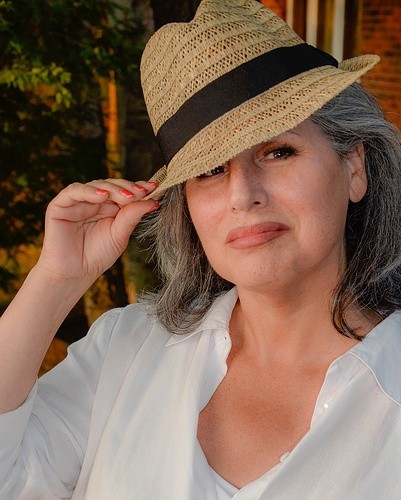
[{"xmin": 0, "ymin": 0, "xmax": 401, "ymax": 500}]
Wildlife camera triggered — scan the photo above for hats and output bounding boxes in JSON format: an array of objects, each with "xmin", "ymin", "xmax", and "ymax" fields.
[{"xmin": 139, "ymin": 1, "xmax": 381, "ymax": 201}]
